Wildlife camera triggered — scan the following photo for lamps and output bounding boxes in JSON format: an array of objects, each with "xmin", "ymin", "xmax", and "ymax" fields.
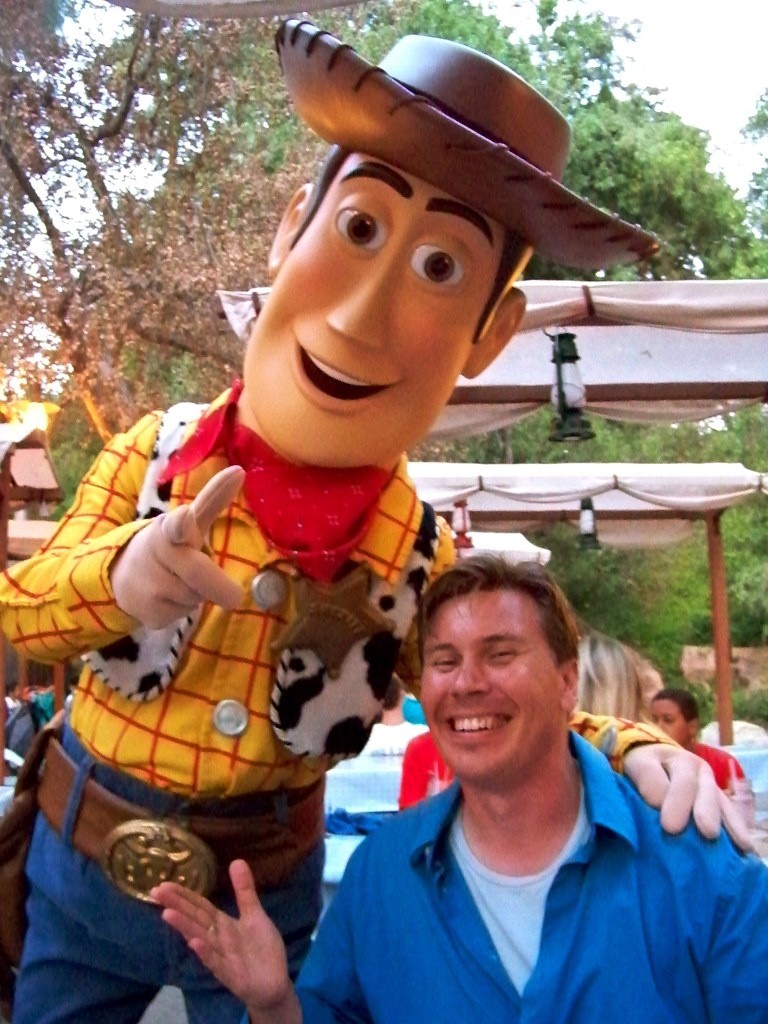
[
  {"xmin": 578, "ymin": 497, "xmax": 602, "ymax": 551},
  {"xmin": 539, "ymin": 326, "xmax": 596, "ymax": 445},
  {"xmin": 453, "ymin": 499, "xmax": 474, "ymax": 549}
]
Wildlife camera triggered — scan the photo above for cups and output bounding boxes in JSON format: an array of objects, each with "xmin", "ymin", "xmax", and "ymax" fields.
[{"xmin": 725, "ymin": 779, "xmax": 754, "ymax": 831}]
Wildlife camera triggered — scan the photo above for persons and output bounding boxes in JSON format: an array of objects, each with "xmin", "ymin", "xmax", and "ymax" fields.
[
  {"xmin": 0, "ymin": 19, "xmax": 757, "ymax": 1024},
  {"xmin": 3, "ymin": 672, "xmax": 456, "ymax": 756},
  {"xmin": 651, "ymin": 687, "xmax": 746, "ymax": 794},
  {"xmin": 150, "ymin": 552, "xmax": 768, "ymax": 1024}
]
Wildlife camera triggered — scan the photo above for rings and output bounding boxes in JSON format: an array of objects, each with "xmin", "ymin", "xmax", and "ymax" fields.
[{"xmin": 208, "ymin": 922, "xmax": 216, "ymax": 933}]
